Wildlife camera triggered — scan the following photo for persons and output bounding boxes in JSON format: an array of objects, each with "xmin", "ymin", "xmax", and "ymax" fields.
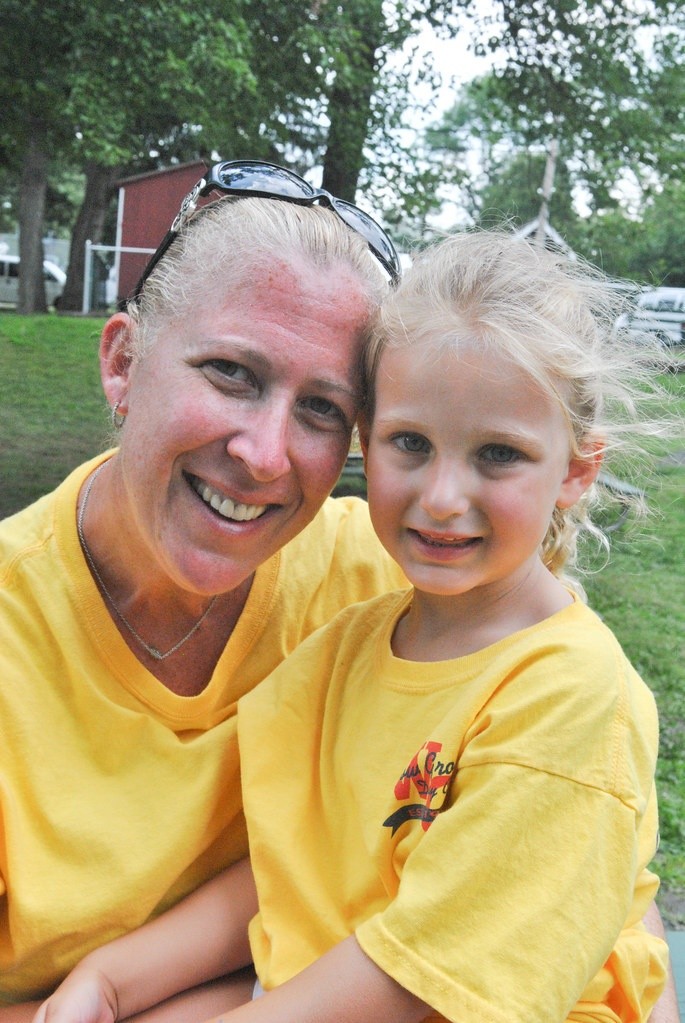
[
  {"xmin": 1, "ymin": 161, "xmax": 680, "ymax": 1023},
  {"xmin": 28, "ymin": 207, "xmax": 682, "ymax": 1023}
]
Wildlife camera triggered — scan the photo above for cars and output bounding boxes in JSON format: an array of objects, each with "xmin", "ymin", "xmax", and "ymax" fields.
[
  {"xmin": 609, "ymin": 286, "xmax": 685, "ymax": 347},
  {"xmin": 0, "ymin": 255, "xmax": 67, "ymax": 308}
]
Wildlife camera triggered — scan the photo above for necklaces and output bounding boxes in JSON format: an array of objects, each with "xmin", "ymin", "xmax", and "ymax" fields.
[{"xmin": 78, "ymin": 460, "xmax": 219, "ymax": 660}]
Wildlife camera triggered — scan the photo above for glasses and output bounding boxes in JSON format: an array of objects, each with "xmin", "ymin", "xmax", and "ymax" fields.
[{"xmin": 137, "ymin": 158, "xmax": 402, "ymax": 330}]
